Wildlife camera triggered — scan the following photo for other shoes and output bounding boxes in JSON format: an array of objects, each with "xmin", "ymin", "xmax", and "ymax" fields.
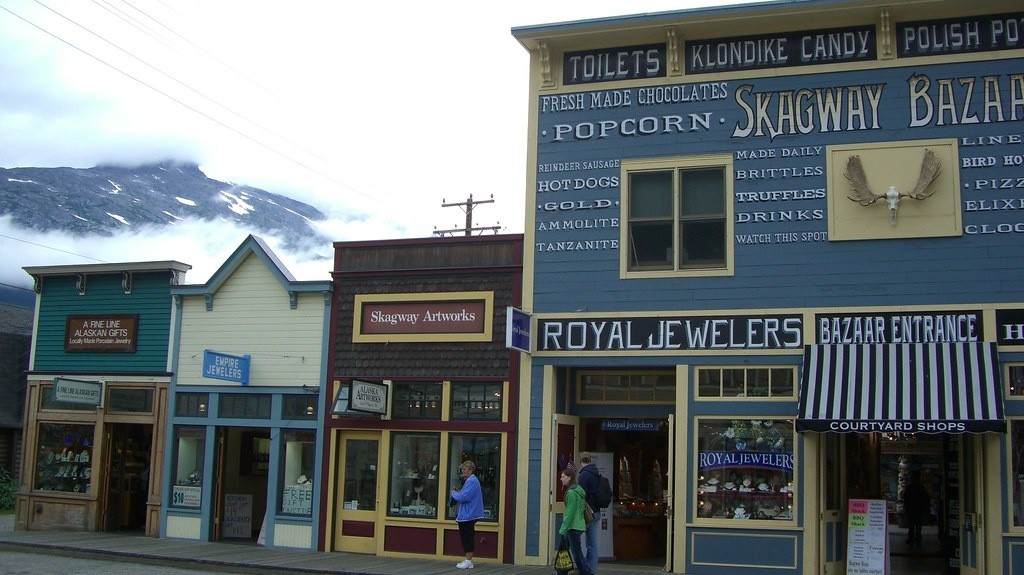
[
  {"xmin": 456, "ymin": 559, "xmax": 475, "ymax": 569},
  {"xmin": 906, "ymin": 539, "xmax": 914, "ymax": 546},
  {"xmin": 916, "ymin": 542, "xmax": 921, "ymax": 547}
]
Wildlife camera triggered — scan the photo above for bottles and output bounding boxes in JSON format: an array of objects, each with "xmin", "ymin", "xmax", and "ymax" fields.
[{"xmin": 38, "ymin": 467, "xmax": 44, "ymax": 477}]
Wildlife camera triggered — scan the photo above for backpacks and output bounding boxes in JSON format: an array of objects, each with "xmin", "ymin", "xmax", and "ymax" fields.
[{"xmin": 596, "ymin": 473, "xmax": 613, "ymax": 508}]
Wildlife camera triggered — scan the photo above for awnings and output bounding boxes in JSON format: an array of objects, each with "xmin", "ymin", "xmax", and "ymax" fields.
[{"xmin": 795, "ymin": 341, "xmax": 1007, "ymax": 435}]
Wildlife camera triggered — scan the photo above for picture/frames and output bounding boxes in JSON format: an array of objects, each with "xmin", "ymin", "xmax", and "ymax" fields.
[{"xmin": 401, "ymin": 505, "xmax": 426, "ymax": 515}]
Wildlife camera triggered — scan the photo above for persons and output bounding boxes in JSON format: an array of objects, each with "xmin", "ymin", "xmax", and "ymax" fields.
[
  {"xmin": 574, "ymin": 452, "xmax": 600, "ymax": 575},
  {"xmin": 555, "ymin": 468, "xmax": 595, "ymax": 575},
  {"xmin": 902, "ymin": 470, "xmax": 932, "ymax": 548},
  {"xmin": 451, "ymin": 460, "xmax": 484, "ymax": 568}
]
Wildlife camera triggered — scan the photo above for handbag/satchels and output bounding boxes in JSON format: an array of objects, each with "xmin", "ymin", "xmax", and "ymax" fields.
[
  {"xmin": 554, "ymin": 535, "xmax": 574, "ymax": 571},
  {"xmin": 584, "ymin": 501, "xmax": 596, "ymax": 524}
]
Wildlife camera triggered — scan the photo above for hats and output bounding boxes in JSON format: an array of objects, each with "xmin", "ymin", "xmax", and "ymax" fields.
[{"xmin": 579, "ymin": 452, "xmax": 591, "ymax": 461}]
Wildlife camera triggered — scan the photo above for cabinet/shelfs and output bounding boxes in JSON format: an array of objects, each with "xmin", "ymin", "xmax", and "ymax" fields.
[
  {"xmin": 34, "ymin": 422, "xmax": 141, "ymax": 529},
  {"xmin": 698, "ymin": 464, "xmax": 792, "ymax": 520}
]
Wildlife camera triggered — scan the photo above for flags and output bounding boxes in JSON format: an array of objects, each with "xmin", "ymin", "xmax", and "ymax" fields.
[{"xmin": 566, "ymin": 457, "xmax": 573, "ymax": 469}]
